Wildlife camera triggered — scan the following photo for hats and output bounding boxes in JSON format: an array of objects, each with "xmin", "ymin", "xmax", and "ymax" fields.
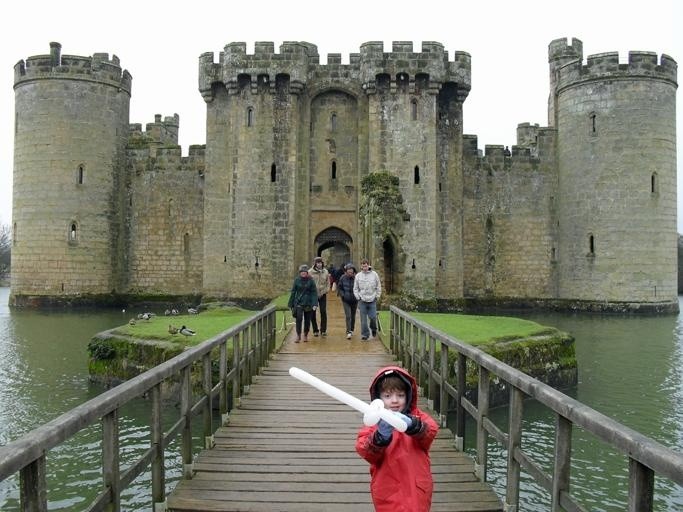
[
  {"xmin": 344, "ymin": 263, "xmax": 358, "ymax": 274},
  {"xmin": 313, "ymin": 257, "xmax": 324, "ymax": 267},
  {"xmin": 299, "ymin": 265, "xmax": 309, "ymax": 272}
]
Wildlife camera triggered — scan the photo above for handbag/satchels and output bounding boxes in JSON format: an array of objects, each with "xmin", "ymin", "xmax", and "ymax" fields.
[{"xmin": 291, "ymin": 300, "xmax": 297, "ymax": 317}]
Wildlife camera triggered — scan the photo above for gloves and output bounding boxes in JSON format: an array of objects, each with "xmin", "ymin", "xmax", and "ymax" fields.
[
  {"xmin": 377, "ymin": 420, "xmax": 393, "ymax": 441},
  {"xmin": 393, "ymin": 411, "xmax": 413, "ymax": 429},
  {"xmin": 313, "ymin": 306, "xmax": 317, "ymax": 310}
]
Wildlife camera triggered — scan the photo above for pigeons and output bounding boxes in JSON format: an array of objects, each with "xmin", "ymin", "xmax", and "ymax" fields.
[
  {"xmin": 129, "ymin": 318, "xmax": 136, "ymax": 325},
  {"xmin": 137, "ymin": 312, "xmax": 157, "ymax": 320},
  {"xmin": 164, "ymin": 309, "xmax": 171, "ymax": 316},
  {"xmin": 172, "ymin": 309, "xmax": 179, "ymax": 314},
  {"xmin": 167, "ymin": 324, "xmax": 181, "ymax": 336},
  {"xmin": 180, "ymin": 325, "xmax": 196, "ymax": 339},
  {"xmin": 187, "ymin": 305, "xmax": 201, "ymax": 316}
]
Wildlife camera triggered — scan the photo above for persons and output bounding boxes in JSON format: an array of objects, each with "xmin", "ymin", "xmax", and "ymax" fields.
[
  {"xmin": 336, "ymin": 265, "xmax": 345, "ymax": 296},
  {"xmin": 287, "ymin": 264, "xmax": 317, "ymax": 343},
  {"xmin": 352, "ymin": 259, "xmax": 380, "ymax": 341},
  {"xmin": 338, "ymin": 264, "xmax": 358, "ymax": 340},
  {"xmin": 326, "ymin": 263, "xmax": 334, "ymax": 291},
  {"xmin": 355, "ymin": 365, "xmax": 440, "ymax": 512},
  {"xmin": 308, "ymin": 257, "xmax": 329, "ymax": 336}
]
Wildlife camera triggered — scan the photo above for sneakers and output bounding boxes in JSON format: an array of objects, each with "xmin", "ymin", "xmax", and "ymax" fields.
[
  {"xmin": 294, "ymin": 336, "xmax": 301, "ymax": 343},
  {"xmin": 346, "ymin": 331, "xmax": 353, "ymax": 339},
  {"xmin": 314, "ymin": 331, "xmax": 319, "ymax": 336},
  {"xmin": 303, "ymin": 336, "xmax": 307, "ymax": 342},
  {"xmin": 322, "ymin": 331, "xmax": 327, "ymax": 337}
]
